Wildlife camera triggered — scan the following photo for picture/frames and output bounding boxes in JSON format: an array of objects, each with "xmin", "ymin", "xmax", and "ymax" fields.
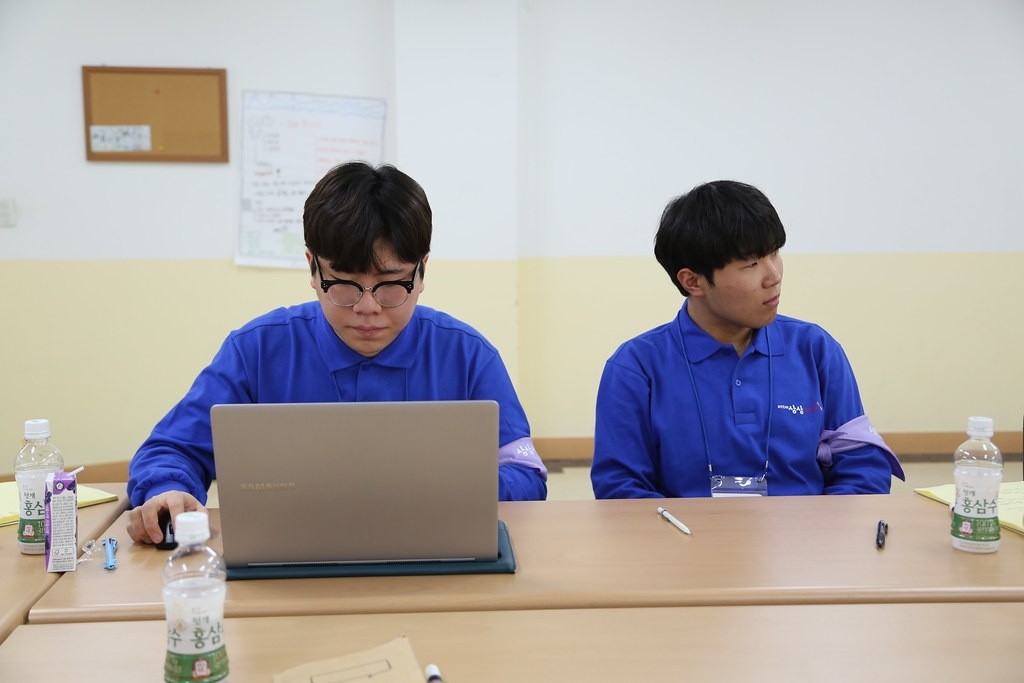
[{"xmin": 81, "ymin": 66, "xmax": 230, "ymax": 164}]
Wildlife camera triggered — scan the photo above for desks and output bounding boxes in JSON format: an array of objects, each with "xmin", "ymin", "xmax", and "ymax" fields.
[{"xmin": 0, "ymin": 483, "xmax": 1024, "ymax": 683}]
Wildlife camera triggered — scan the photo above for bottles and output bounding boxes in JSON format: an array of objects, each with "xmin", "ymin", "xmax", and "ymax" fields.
[
  {"xmin": 15, "ymin": 418, "xmax": 64, "ymax": 554},
  {"xmin": 161, "ymin": 512, "xmax": 230, "ymax": 683},
  {"xmin": 951, "ymin": 417, "xmax": 1003, "ymax": 553}
]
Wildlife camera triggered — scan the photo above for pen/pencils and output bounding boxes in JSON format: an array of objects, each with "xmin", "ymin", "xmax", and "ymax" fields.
[
  {"xmin": 102, "ymin": 537, "xmax": 117, "ymax": 570},
  {"xmin": 657, "ymin": 506, "xmax": 691, "ymax": 534},
  {"xmin": 876, "ymin": 519, "xmax": 888, "ymax": 547}
]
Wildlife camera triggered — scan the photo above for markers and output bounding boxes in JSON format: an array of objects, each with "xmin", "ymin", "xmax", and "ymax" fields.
[{"xmin": 425, "ymin": 663, "xmax": 443, "ymax": 683}]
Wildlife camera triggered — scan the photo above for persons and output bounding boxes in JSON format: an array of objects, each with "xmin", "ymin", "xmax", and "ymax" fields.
[
  {"xmin": 589, "ymin": 181, "xmax": 905, "ymax": 499},
  {"xmin": 126, "ymin": 162, "xmax": 547, "ymax": 546}
]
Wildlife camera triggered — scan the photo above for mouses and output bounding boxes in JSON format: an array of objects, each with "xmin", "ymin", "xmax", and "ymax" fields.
[{"xmin": 155, "ymin": 512, "xmax": 178, "ymax": 550}]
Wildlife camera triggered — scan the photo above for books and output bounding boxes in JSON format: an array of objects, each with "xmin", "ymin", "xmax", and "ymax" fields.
[
  {"xmin": 0, "ymin": 482, "xmax": 118, "ymax": 528},
  {"xmin": 271, "ymin": 637, "xmax": 428, "ymax": 683}
]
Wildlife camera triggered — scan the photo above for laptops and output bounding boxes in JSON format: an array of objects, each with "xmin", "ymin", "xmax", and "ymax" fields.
[{"xmin": 210, "ymin": 401, "xmax": 499, "ymax": 569}]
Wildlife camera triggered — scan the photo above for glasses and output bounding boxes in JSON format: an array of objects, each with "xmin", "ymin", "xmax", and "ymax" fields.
[{"xmin": 314, "ymin": 253, "xmax": 420, "ymax": 308}]
